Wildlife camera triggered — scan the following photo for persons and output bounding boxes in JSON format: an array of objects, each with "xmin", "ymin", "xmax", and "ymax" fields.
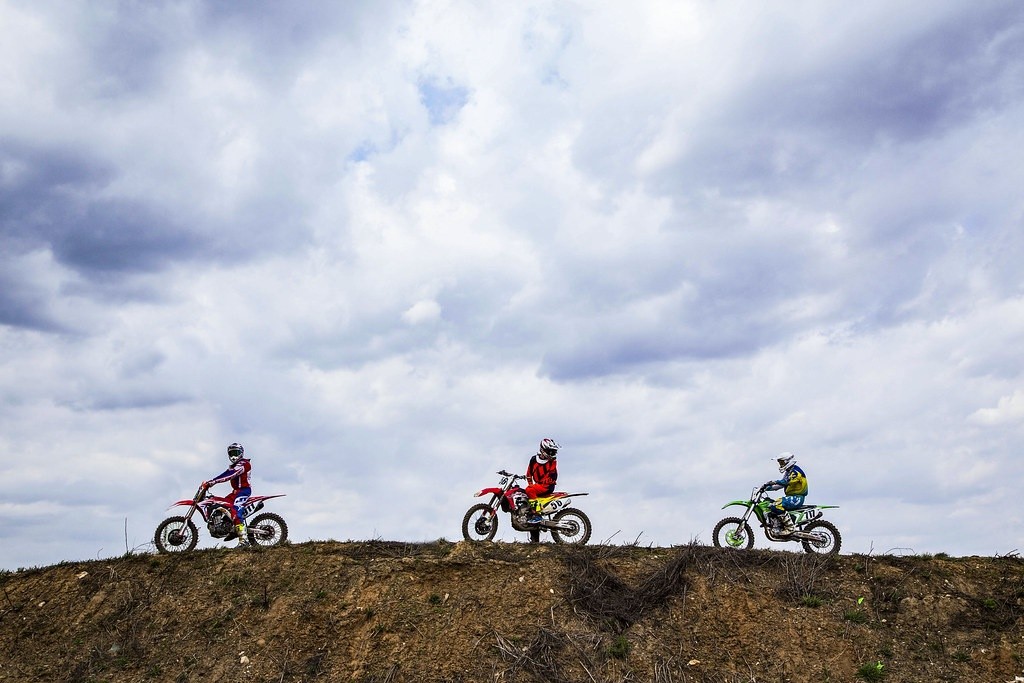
[
  {"xmin": 760, "ymin": 451, "xmax": 808, "ymax": 536},
  {"xmin": 202, "ymin": 442, "xmax": 253, "ymax": 548},
  {"xmin": 525, "ymin": 437, "xmax": 558, "ymax": 544}
]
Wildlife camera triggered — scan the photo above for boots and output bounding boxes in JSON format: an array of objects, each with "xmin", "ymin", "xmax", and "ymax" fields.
[
  {"xmin": 779, "ymin": 511, "xmax": 795, "ymax": 535},
  {"xmin": 235, "ymin": 524, "xmax": 251, "ymax": 550},
  {"xmin": 526, "ymin": 499, "xmax": 543, "ymax": 524}
]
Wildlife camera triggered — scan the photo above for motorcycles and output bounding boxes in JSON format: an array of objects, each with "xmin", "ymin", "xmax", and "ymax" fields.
[
  {"xmin": 711, "ymin": 481, "xmax": 843, "ymax": 556},
  {"xmin": 462, "ymin": 469, "xmax": 593, "ymax": 545},
  {"xmin": 153, "ymin": 481, "xmax": 289, "ymax": 556}
]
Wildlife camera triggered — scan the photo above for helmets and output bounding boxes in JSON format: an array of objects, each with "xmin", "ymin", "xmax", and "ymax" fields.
[
  {"xmin": 228, "ymin": 443, "xmax": 244, "ymax": 463},
  {"xmin": 771, "ymin": 452, "xmax": 797, "ymax": 473},
  {"xmin": 540, "ymin": 439, "xmax": 558, "ymax": 458}
]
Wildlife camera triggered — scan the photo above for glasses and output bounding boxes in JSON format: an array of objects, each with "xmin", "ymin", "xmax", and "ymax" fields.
[
  {"xmin": 541, "ymin": 444, "xmax": 557, "ymax": 456},
  {"xmin": 779, "ymin": 461, "xmax": 788, "ymax": 466},
  {"xmin": 229, "ymin": 450, "xmax": 243, "ymax": 457}
]
